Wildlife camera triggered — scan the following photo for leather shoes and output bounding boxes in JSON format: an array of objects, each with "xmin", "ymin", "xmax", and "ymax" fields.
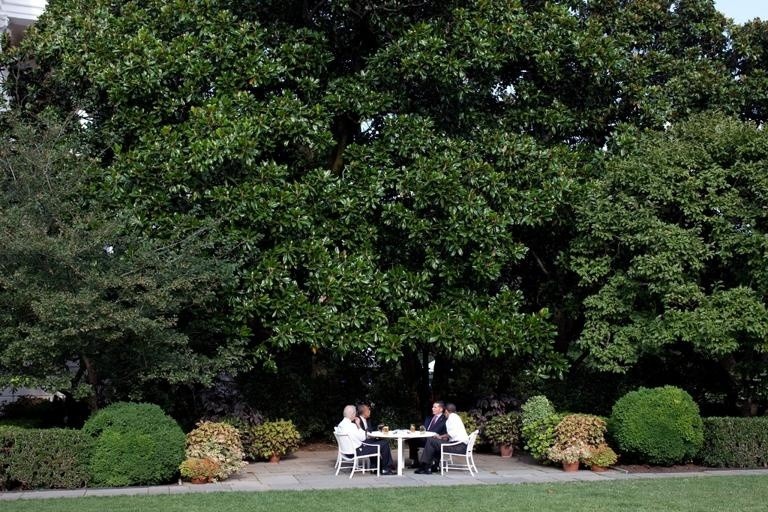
[
  {"xmin": 369, "ymin": 463, "xmax": 397, "ymax": 476},
  {"xmin": 408, "ymin": 460, "xmax": 440, "ymax": 474}
]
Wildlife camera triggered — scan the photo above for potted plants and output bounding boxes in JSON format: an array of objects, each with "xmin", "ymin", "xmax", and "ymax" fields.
[
  {"xmin": 488, "ymin": 390, "xmax": 619, "ymax": 475},
  {"xmin": 173, "ymin": 413, "xmax": 309, "ymax": 486}
]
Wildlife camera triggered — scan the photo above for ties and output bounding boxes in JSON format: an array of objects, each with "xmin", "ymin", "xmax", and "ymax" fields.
[{"xmin": 428, "ymin": 415, "xmax": 438, "ymax": 431}]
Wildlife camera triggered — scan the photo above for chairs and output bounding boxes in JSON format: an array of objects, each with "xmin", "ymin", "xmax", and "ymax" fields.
[{"xmin": 332, "ymin": 416, "xmax": 480, "ymax": 477}]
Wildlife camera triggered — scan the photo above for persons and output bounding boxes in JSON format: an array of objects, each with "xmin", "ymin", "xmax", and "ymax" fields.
[
  {"xmin": 408, "ymin": 401, "xmax": 469, "ymax": 474},
  {"xmin": 338, "ymin": 405, "xmax": 397, "ymax": 475}
]
